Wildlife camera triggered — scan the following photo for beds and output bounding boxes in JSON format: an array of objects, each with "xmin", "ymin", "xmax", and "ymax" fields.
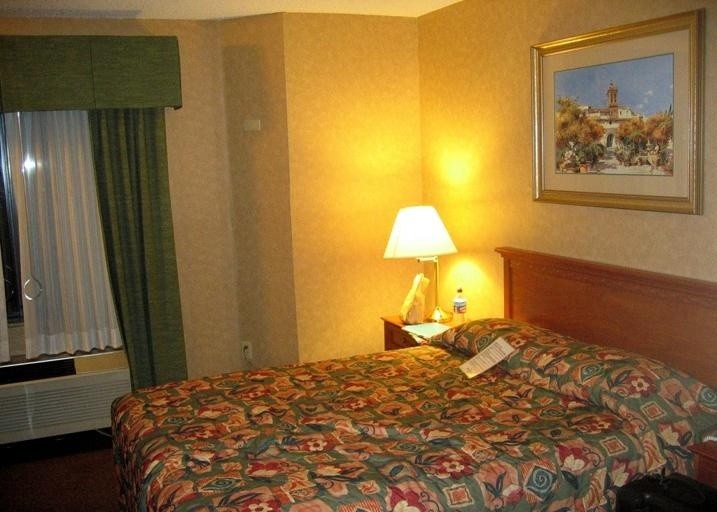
[{"xmin": 112, "ymin": 248, "xmax": 717, "ymax": 512}]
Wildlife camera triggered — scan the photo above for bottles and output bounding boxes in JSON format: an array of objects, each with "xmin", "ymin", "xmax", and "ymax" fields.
[{"xmin": 453, "ymin": 288, "xmax": 466, "ymax": 326}]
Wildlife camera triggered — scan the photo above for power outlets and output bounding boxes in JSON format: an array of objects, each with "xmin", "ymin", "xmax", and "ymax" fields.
[{"xmin": 239, "ymin": 341, "xmax": 252, "ymax": 360}]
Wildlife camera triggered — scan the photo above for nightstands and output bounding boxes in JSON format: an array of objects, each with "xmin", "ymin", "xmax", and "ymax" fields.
[
  {"xmin": 379, "ymin": 313, "xmax": 458, "ymax": 350},
  {"xmin": 689, "ymin": 437, "xmax": 717, "ymax": 488}
]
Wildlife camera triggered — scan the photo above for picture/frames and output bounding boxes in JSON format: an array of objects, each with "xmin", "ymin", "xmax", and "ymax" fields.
[{"xmin": 529, "ymin": 7, "xmax": 702, "ymax": 215}]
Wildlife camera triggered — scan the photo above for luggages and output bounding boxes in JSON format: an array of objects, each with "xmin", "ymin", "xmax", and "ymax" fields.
[{"xmin": 615, "ymin": 473, "xmax": 716, "ymax": 511}]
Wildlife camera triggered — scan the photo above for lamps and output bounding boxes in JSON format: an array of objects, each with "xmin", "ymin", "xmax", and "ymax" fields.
[{"xmin": 385, "ymin": 205, "xmax": 458, "ymax": 322}]
